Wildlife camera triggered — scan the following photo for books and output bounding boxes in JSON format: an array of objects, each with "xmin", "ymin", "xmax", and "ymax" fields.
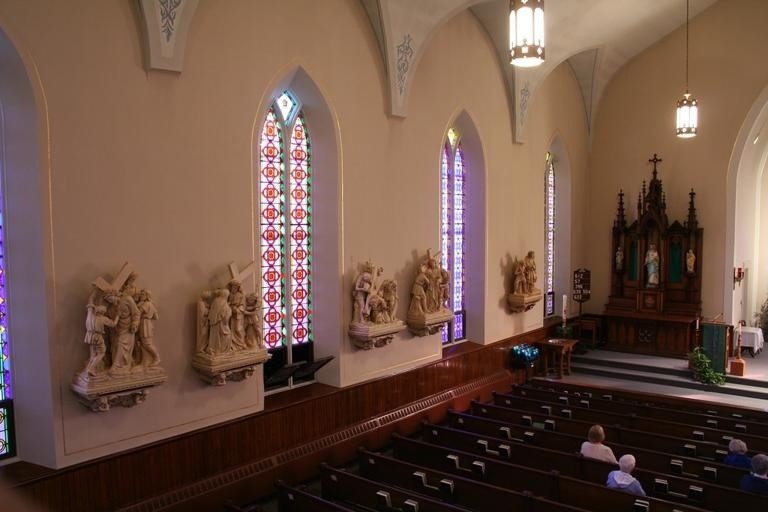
[
  {"xmin": 376, "ymin": 389, "xmax": 613, "ymax": 512},
  {"xmin": 633, "ymin": 409, "xmax": 747, "ymax": 511}
]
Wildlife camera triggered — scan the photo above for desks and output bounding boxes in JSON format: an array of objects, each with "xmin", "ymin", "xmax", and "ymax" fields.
[
  {"xmin": 539, "ymin": 335, "xmax": 579, "ymax": 378},
  {"xmin": 575, "ymin": 316, "xmax": 602, "ymax": 351},
  {"xmin": 736, "ymin": 326, "xmax": 761, "ymax": 358}
]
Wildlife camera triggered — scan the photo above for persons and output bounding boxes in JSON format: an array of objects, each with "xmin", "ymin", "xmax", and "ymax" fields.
[
  {"xmin": 616, "ymin": 246, "xmax": 624, "ymax": 271},
  {"xmin": 512, "ymin": 259, "xmax": 529, "ymax": 293},
  {"xmin": 605, "ymin": 454, "xmax": 648, "ymax": 498},
  {"xmin": 374, "ymin": 278, "xmax": 399, "ymax": 321},
  {"xmin": 120, "ymin": 270, "xmax": 142, "ymax": 297},
  {"xmin": 360, "ymin": 286, "xmax": 392, "ymax": 324},
  {"xmin": 580, "ymin": 424, "xmax": 619, "ymax": 466},
  {"xmin": 194, "ymin": 289, "xmax": 214, "ymax": 354},
  {"xmin": 78, "ymin": 290, "xmax": 121, "ymax": 378},
  {"xmin": 205, "ymin": 286, "xmax": 233, "ymax": 355},
  {"xmin": 348, "ymin": 259, "xmax": 378, "ymax": 321},
  {"xmin": 223, "ymin": 278, "xmax": 248, "ymax": 352},
  {"xmin": 129, "ymin": 287, "xmax": 161, "ymax": 370},
  {"xmin": 411, "ymin": 269, "xmax": 435, "ymax": 313},
  {"xmin": 723, "ymin": 438, "xmax": 754, "ymax": 469},
  {"xmin": 99, "ymin": 284, "xmax": 141, "ymax": 375},
  {"xmin": 364, "ymin": 264, "xmax": 386, "ymax": 293},
  {"xmin": 737, "ymin": 453, "xmax": 768, "ymax": 496},
  {"xmin": 684, "ymin": 248, "xmax": 697, "ymax": 274},
  {"xmin": 522, "ymin": 250, "xmax": 538, "ymax": 294},
  {"xmin": 424, "ymin": 255, "xmax": 443, "ymax": 310},
  {"xmin": 243, "ymin": 291, "xmax": 267, "ymax": 351},
  {"xmin": 641, "ymin": 242, "xmax": 661, "ymax": 288},
  {"xmin": 437, "ymin": 268, "xmax": 450, "ymax": 308}
]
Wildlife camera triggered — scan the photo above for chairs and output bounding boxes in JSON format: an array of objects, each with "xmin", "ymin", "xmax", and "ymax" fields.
[{"xmin": 737, "ymin": 319, "xmax": 763, "ymax": 354}]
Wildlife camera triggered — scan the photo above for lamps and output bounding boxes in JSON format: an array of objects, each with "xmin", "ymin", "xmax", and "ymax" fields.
[{"xmin": 508, "ymin": 0, "xmax": 701, "ymax": 139}]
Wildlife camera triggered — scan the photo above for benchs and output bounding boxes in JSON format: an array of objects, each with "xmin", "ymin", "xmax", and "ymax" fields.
[{"xmin": 237, "ymin": 378, "xmax": 768, "ymax": 511}]
